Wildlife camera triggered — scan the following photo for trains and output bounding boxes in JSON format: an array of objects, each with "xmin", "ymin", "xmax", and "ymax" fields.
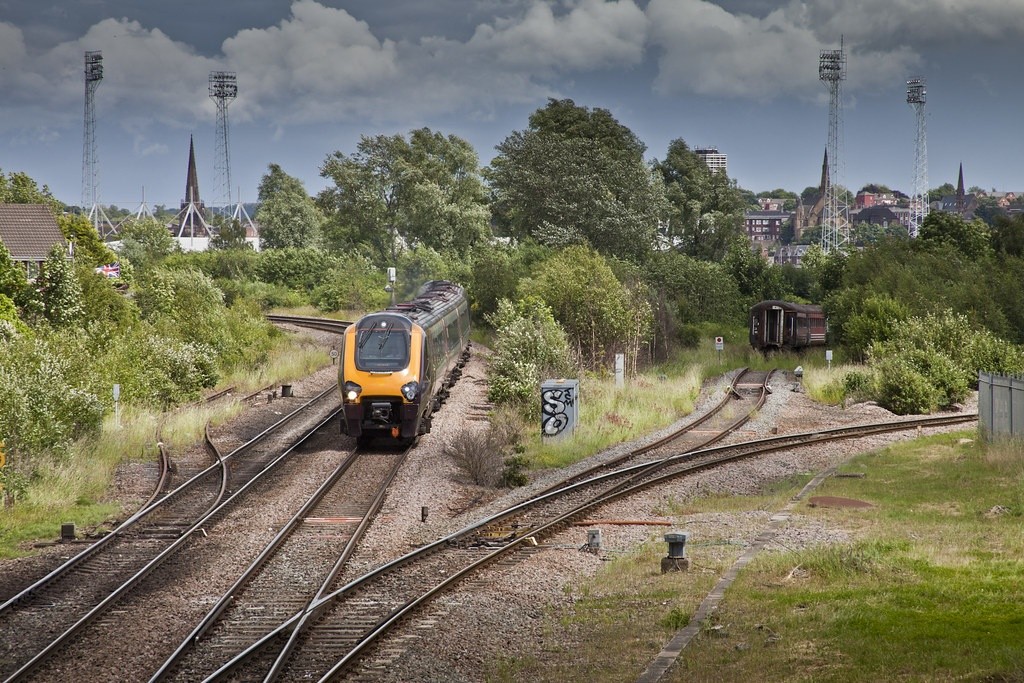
[
  {"xmin": 337, "ymin": 277, "xmax": 471, "ymax": 449},
  {"xmin": 748, "ymin": 300, "xmax": 834, "ymax": 351}
]
